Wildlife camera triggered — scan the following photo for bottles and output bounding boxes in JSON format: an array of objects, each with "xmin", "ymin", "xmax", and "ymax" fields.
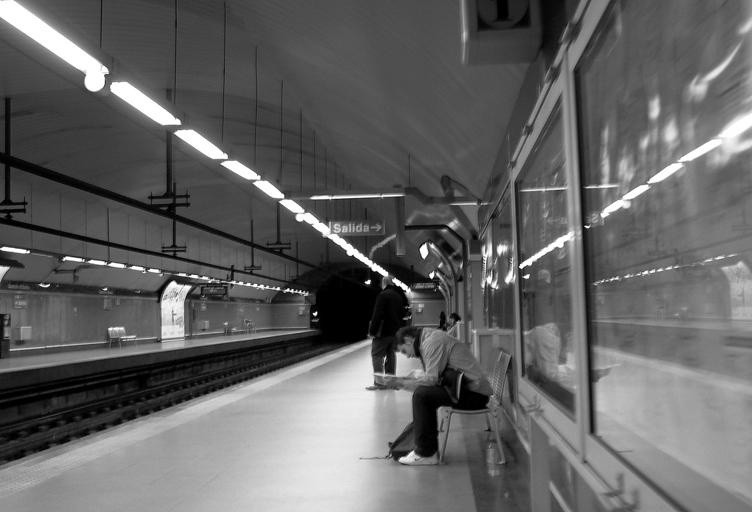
[{"xmin": 484, "ymin": 430, "xmax": 497, "ymax": 468}]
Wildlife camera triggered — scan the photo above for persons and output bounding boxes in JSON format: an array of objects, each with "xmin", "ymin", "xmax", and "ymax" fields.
[
  {"xmin": 440, "ymin": 312, "xmax": 445, "ymax": 328},
  {"xmin": 367, "ymin": 276, "xmax": 409, "ymax": 390},
  {"xmin": 389, "ymin": 327, "xmax": 492, "ymax": 466},
  {"xmin": 447, "ymin": 313, "xmax": 462, "ymax": 337},
  {"xmin": 522, "ymin": 319, "xmax": 611, "ymax": 385}
]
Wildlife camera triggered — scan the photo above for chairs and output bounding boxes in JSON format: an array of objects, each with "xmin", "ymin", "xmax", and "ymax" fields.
[{"xmin": 433, "ymin": 347, "xmax": 513, "ymax": 463}]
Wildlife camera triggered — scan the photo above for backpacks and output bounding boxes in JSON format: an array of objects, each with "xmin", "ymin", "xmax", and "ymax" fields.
[{"xmin": 358, "ymin": 418, "xmax": 418, "ymax": 462}]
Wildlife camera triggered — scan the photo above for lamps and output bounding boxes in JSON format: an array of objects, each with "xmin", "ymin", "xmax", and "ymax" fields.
[
  {"xmin": 2, "ymin": 166, "xmax": 222, "ymax": 283},
  {"xmin": 109, "ymin": 4, "xmax": 182, "ymax": 126},
  {"xmin": 2, "ymin": 1, "xmax": 112, "ymax": 93},
  {"xmin": 171, "ymin": 3, "xmax": 228, "ymax": 160},
  {"xmin": 222, "ymin": 47, "xmax": 413, "ymax": 297}
]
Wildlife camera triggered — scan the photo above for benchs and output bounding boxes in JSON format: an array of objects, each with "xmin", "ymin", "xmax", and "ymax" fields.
[
  {"xmin": 105, "ymin": 325, "xmax": 138, "ymax": 348},
  {"xmin": 221, "ymin": 318, "xmax": 259, "ymax": 337}
]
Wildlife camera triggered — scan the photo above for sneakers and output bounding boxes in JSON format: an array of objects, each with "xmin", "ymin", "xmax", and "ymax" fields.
[
  {"xmin": 397, "ymin": 448, "xmax": 443, "ymax": 467},
  {"xmin": 364, "ymin": 384, "xmax": 387, "ymax": 391}
]
